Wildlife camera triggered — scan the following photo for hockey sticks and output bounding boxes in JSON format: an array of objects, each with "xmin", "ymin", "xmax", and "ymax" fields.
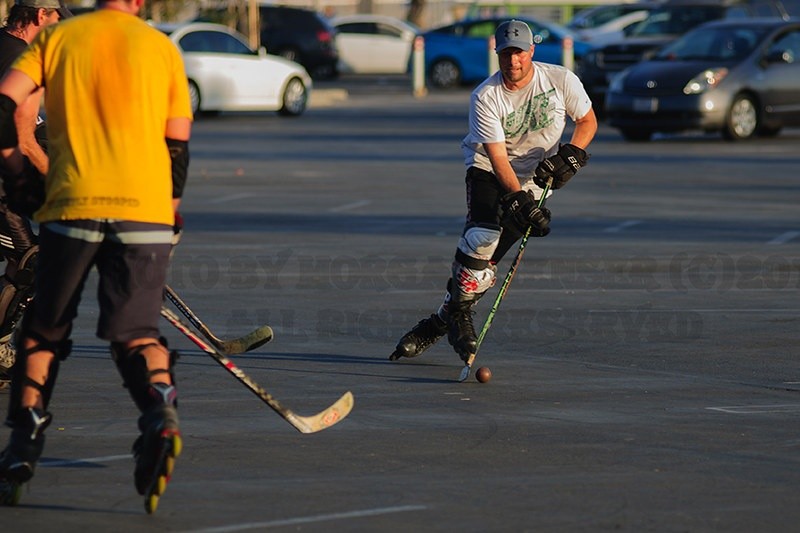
[
  {"xmin": 163, "ymin": 284, "xmax": 274, "ymax": 355},
  {"xmin": 157, "ymin": 304, "xmax": 355, "ymax": 433},
  {"xmin": 458, "ymin": 177, "xmax": 554, "ymax": 383}
]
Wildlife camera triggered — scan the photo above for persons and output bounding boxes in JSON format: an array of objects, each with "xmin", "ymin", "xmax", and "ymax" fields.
[
  {"xmin": 0, "ymin": 0, "xmax": 63, "ymax": 391},
  {"xmin": 390, "ymin": 20, "xmax": 598, "ymax": 362},
  {"xmin": 0, "ymin": 0, "xmax": 193, "ymax": 516}
]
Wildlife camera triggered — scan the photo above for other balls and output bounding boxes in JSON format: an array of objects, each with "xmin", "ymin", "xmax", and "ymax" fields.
[{"xmin": 475, "ymin": 367, "xmax": 492, "ymax": 383}]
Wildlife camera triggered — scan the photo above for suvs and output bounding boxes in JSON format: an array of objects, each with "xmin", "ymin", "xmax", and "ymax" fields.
[{"xmin": 190, "ymin": 4, "xmax": 340, "ymax": 82}]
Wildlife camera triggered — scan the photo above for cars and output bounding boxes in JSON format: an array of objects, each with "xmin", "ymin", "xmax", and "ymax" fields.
[
  {"xmin": 145, "ymin": 19, "xmax": 314, "ymax": 118},
  {"xmin": 562, "ymin": 0, "xmax": 800, "ymax": 84},
  {"xmin": 607, "ymin": 14, "xmax": 800, "ymax": 144},
  {"xmin": 326, "ymin": 12, "xmax": 420, "ymax": 76},
  {"xmin": 408, "ymin": 12, "xmax": 597, "ymax": 92}
]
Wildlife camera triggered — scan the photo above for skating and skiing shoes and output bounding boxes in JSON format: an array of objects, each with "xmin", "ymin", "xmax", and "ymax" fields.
[
  {"xmin": 0, "ymin": 326, "xmax": 18, "ymax": 390},
  {"xmin": 445, "ymin": 300, "xmax": 479, "ymax": 367},
  {"xmin": 130, "ymin": 382, "xmax": 182, "ymax": 514},
  {"xmin": 1, "ymin": 406, "xmax": 53, "ymax": 505},
  {"xmin": 388, "ymin": 313, "xmax": 447, "ymax": 361}
]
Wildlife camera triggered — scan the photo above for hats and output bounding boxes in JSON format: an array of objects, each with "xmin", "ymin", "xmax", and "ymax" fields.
[
  {"xmin": 495, "ymin": 19, "xmax": 534, "ymax": 54},
  {"xmin": 15, "ymin": 0, "xmax": 74, "ymax": 20}
]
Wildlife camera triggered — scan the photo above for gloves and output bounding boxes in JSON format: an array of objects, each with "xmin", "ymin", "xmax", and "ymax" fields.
[
  {"xmin": 500, "ymin": 190, "xmax": 552, "ymax": 237},
  {"xmin": 533, "ymin": 143, "xmax": 592, "ymax": 191}
]
